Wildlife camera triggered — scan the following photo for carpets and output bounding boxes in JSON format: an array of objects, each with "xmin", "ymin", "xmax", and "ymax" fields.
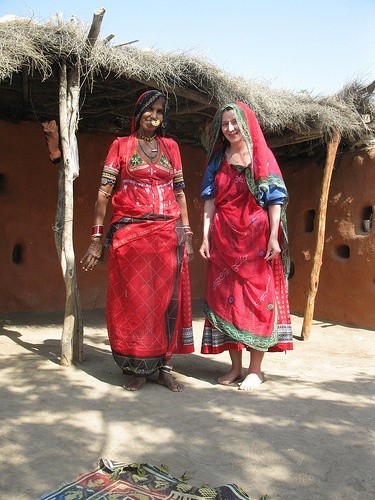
[{"xmin": 33, "ymin": 457, "xmax": 272, "ymax": 500}]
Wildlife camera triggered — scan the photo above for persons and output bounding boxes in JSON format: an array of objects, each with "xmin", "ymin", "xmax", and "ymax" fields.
[
  {"xmin": 80, "ymin": 90, "xmax": 195, "ymax": 392},
  {"xmin": 198, "ymin": 100, "xmax": 294, "ymax": 391}
]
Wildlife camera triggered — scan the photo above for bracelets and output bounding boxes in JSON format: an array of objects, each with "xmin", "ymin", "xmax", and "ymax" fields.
[
  {"xmin": 183, "ymin": 227, "xmax": 194, "ymax": 236},
  {"xmin": 91, "ymin": 224, "xmax": 104, "ymax": 238}
]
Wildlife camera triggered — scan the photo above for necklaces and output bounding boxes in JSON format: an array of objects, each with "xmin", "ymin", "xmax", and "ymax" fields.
[
  {"xmin": 137, "ymin": 130, "xmax": 156, "ymax": 140},
  {"xmin": 229, "ymin": 151, "xmax": 247, "ymax": 163},
  {"xmin": 138, "ymin": 138, "xmax": 159, "ymax": 163}
]
